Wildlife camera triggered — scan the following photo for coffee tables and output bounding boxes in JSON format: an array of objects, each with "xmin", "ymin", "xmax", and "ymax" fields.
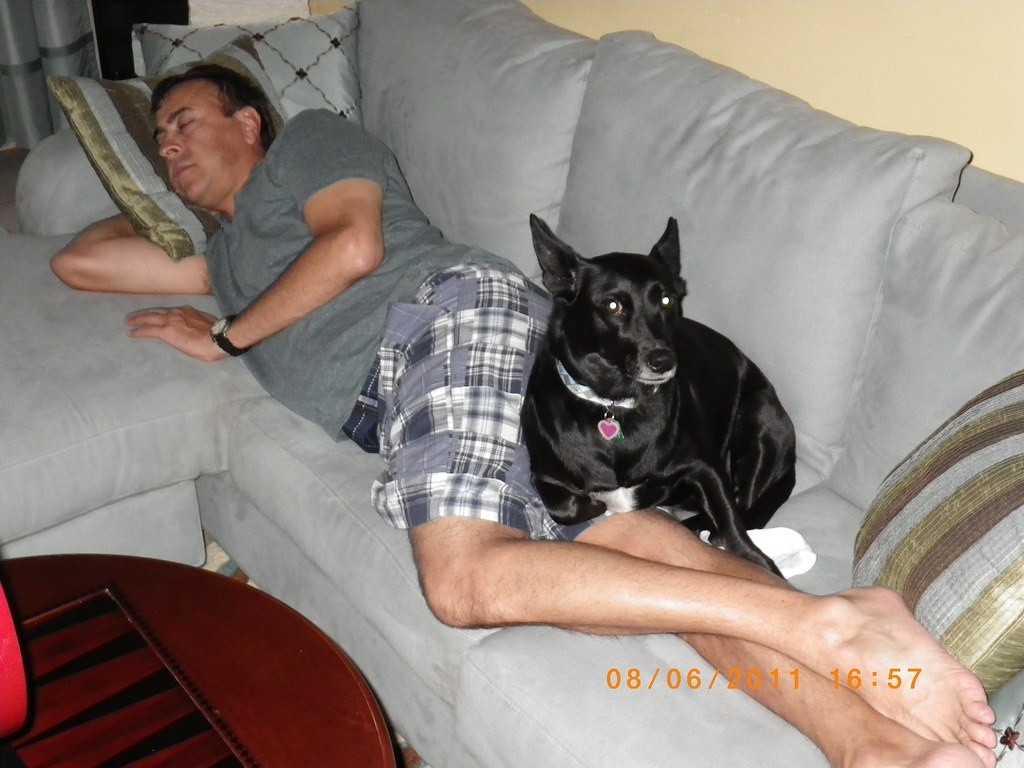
[{"xmin": 1, "ymin": 554, "xmax": 399, "ymax": 768}]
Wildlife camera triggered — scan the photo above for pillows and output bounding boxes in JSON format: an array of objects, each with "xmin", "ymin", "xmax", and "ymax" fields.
[
  {"xmin": 849, "ymin": 368, "xmax": 1024, "ymax": 701},
  {"xmin": 129, "ymin": 2, "xmax": 363, "ymax": 130},
  {"xmin": 46, "ymin": 33, "xmax": 290, "ymax": 262}
]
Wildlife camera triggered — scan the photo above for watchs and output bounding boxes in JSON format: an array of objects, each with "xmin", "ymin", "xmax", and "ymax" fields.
[{"xmin": 208, "ymin": 314, "xmax": 250, "ymax": 358}]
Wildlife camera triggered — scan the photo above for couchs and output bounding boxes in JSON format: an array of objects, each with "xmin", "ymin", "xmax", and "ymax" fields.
[{"xmin": 1, "ymin": 1, "xmax": 1023, "ymax": 768}]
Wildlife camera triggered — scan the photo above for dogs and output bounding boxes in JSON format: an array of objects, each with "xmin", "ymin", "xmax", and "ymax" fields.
[{"xmin": 520, "ymin": 212, "xmax": 818, "ymax": 582}]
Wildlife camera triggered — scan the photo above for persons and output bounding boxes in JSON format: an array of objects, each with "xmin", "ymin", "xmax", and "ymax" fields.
[{"xmin": 51, "ymin": 64, "xmax": 1001, "ymax": 768}]
